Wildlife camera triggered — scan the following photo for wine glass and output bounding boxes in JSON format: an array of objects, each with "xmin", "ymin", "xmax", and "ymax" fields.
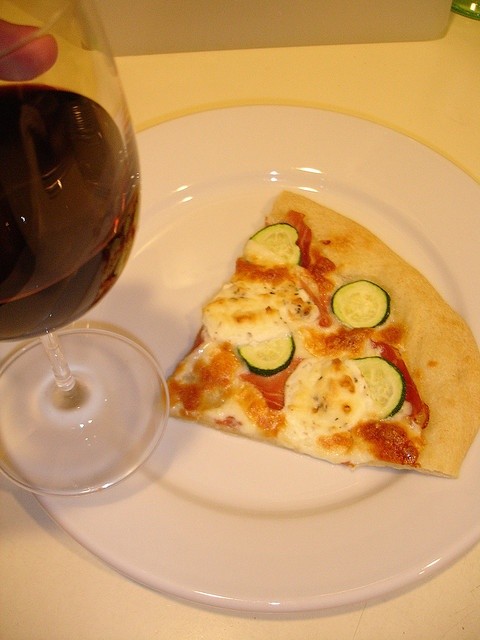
[{"xmin": 0, "ymin": 0, "xmax": 170, "ymax": 498}]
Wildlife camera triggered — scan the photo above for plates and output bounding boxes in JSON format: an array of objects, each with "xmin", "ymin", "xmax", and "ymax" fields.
[{"xmin": 1, "ymin": 104, "xmax": 480, "ymax": 614}]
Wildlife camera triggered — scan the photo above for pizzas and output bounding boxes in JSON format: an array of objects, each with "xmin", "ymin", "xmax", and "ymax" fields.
[{"xmin": 161, "ymin": 190, "xmax": 480, "ymax": 479}]
